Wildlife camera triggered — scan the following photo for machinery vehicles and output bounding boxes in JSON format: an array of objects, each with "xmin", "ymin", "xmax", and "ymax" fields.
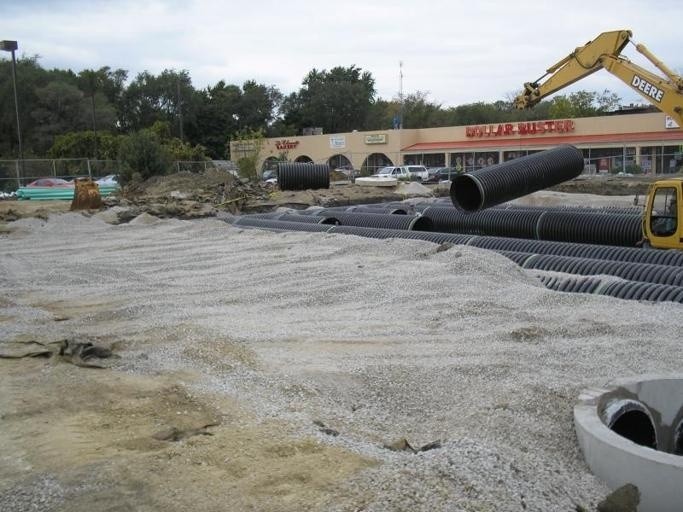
[{"xmin": 514, "ymin": 29, "xmax": 683, "ymax": 250}]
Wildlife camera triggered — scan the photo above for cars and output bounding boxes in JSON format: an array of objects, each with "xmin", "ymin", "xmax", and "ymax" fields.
[
  {"xmin": 370, "ymin": 165, "xmax": 465, "ymax": 181},
  {"xmin": 26, "ymin": 173, "xmax": 120, "ymax": 188}
]
